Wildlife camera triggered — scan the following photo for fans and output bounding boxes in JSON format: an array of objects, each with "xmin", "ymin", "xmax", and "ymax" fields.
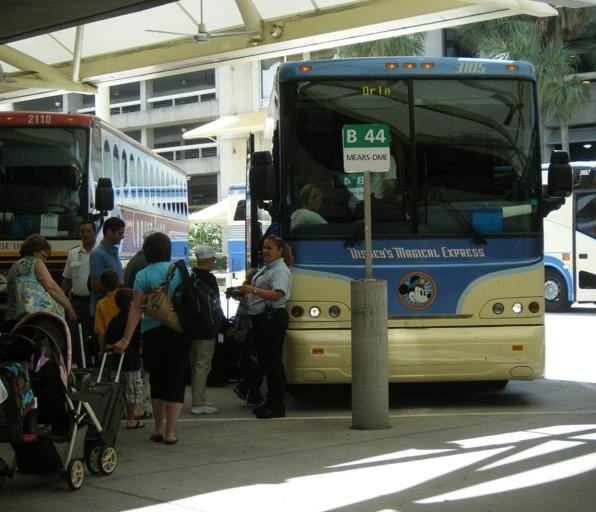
[
  {"xmin": 0, "ymin": 64, "xmax": 38, "ymax": 85},
  {"xmin": 144, "ymin": 0, "xmax": 259, "ymax": 46}
]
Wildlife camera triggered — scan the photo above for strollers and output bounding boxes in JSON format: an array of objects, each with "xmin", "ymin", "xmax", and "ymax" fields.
[{"xmin": 0, "ymin": 307, "xmax": 88, "ymax": 499}]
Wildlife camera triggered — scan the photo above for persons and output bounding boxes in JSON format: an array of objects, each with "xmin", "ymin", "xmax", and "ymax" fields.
[
  {"xmin": 192, "ymin": 246, "xmax": 231, "ymax": 413},
  {"xmin": 113, "ymin": 233, "xmax": 197, "ymax": 443},
  {"xmin": 347, "ymin": 154, "xmax": 398, "ymax": 216},
  {"xmin": 232, "ymin": 235, "xmax": 293, "ymax": 418},
  {"xmin": 90, "ymin": 218, "xmax": 126, "ymax": 356},
  {"xmin": 61, "ymin": 220, "xmax": 99, "ymax": 363},
  {"xmin": 11, "ymin": 234, "xmax": 78, "ymax": 437},
  {"xmin": 291, "ymin": 182, "xmax": 328, "ymax": 232},
  {"xmin": 31, "ymin": 179, "xmax": 80, "ymax": 238},
  {"xmin": 94, "ymin": 249, "xmax": 152, "ymax": 428}
]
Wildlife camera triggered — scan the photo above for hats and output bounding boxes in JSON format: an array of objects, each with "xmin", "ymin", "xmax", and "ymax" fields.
[{"xmin": 194, "ymin": 245, "xmax": 223, "ymax": 260}]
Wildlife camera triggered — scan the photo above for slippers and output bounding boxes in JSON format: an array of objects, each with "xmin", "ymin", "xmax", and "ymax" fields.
[
  {"xmin": 133, "ymin": 410, "xmax": 153, "ymax": 420},
  {"xmin": 164, "ymin": 437, "xmax": 178, "ymax": 445},
  {"xmin": 150, "ymin": 432, "xmax": 164, "ymax": 443},
  {"xmin": 125, "ymin": 421, "xmax": 146, "ymax": 430}
]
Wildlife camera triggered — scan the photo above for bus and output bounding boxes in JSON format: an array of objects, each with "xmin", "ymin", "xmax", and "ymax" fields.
[
  {"xmin": 0, "ymin": 108, "xmax": 190, "ymax": 366},
  {"xmin": 542, "ymin": 160, "xmax": 595, "ymax": 312},
  {"xmin": 226, "ymin": 184, "xmax": 251, "ymax": 285},
  {"xmin": 243, "ymin": 58, "xmax": 571, "ymax": 386}
]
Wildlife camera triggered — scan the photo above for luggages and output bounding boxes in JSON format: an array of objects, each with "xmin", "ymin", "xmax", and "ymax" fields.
[
  {"xmin": 67, "ymin": 368, "xmax": 110, "ymax": 395},
  {"xmin": 75, "ymin": 379, "xmax": 127, "ymax": 445}
]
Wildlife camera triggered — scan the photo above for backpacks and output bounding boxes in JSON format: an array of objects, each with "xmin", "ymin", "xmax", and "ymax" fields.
[{"xmin": 177, "ymin": 272, "xmax": 224, "ymax": 342}]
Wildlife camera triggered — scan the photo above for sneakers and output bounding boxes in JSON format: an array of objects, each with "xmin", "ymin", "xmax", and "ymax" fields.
[
  {"xmin": 190, "ymin": 405, "xmax": 219, "ymax": 415},
  {"xmin": 232, "ymin": 381, "xmax": 248, "ymax": 400},
  {"xmin": 246, "ymin": 397, "xmax": 267, "ymax": 408}
]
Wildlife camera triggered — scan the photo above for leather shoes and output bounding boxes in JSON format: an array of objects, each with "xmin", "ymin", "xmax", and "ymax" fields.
[{"xmin": 252, "ymin": 403, "xmax": 286, "ymax": 419}]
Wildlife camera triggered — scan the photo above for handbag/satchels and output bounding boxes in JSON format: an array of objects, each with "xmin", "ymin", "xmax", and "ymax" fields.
[
  {"xmin": 13, "ymin": 274, "xmax": 67, "ymax": 323},
  {"xmin": 139, "ymin": 283, "xmax": 187, "ymax": 336}
]
